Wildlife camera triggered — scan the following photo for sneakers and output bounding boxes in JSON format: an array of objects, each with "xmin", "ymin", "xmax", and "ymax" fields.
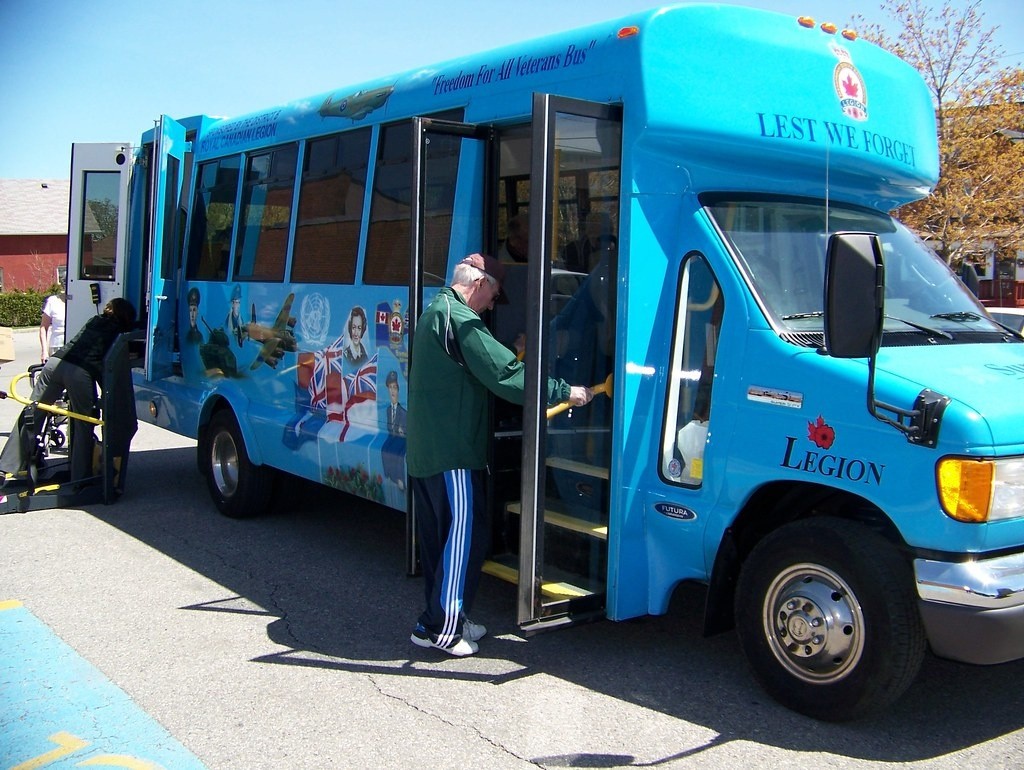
[
  {"xmin": 463, "ymin": 618, "xmax": 488, "ymax": 641},
  {"xmin": 410, "ymin": 621, "xmax": 479, "ymax": 656}
]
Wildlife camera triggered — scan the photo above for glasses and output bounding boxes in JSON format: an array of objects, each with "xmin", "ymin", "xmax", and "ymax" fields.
[{"xmin": 474, "ymin": 278, "xmax": 502, "ymax": 303}]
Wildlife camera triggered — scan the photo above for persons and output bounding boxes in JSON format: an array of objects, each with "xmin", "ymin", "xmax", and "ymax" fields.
[
  {"xmin": 961, "ymin": 254, "xmax": 980, "ymax": 296},
  {"xmin": 0, "ymin": 271, "xmax": 138, "ymax": 487},
  {"xmin": 411, "ymin": 211, "xmax": 618, "ymax": 657}
]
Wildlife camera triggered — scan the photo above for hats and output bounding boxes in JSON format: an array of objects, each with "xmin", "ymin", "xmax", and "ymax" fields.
[
  {"xmin": 586, "ymin": 211, "xmax": 618, "ymax": 244},
  {"xmin": 187, "ymin": 288, "xmax": 201, "ymax": 307},
  {"xmin": 386, "ymin": 371, "xmax": 397, "ymax": 386},
  {"xmin": 461, "ymin": 253, "xmax": 511, "ymax": 306},
  {"xmin": 230, "ymin": 284, "xmax": 242, "ymax": 302}
]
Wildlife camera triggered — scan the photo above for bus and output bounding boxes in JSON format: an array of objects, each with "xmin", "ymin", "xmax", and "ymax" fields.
[{"xmin": 62, "ymin": 1, "xmax": 1024, "ymax": 731}]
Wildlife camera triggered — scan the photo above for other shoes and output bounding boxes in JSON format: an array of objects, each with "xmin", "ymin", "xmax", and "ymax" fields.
[{"xmin": 72, "ymin": 483, "xmax": 94, "ymax": 494}]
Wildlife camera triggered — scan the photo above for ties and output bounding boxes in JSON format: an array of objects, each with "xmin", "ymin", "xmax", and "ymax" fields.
[{"xmin": 393, "ymin": 406, "xmax": 395, "ymax": 421}]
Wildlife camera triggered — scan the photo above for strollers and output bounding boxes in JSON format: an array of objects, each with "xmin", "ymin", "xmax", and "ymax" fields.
[{"xmin": 28, "ymin": 361, "xmax": 73, "ymax": 456}]
